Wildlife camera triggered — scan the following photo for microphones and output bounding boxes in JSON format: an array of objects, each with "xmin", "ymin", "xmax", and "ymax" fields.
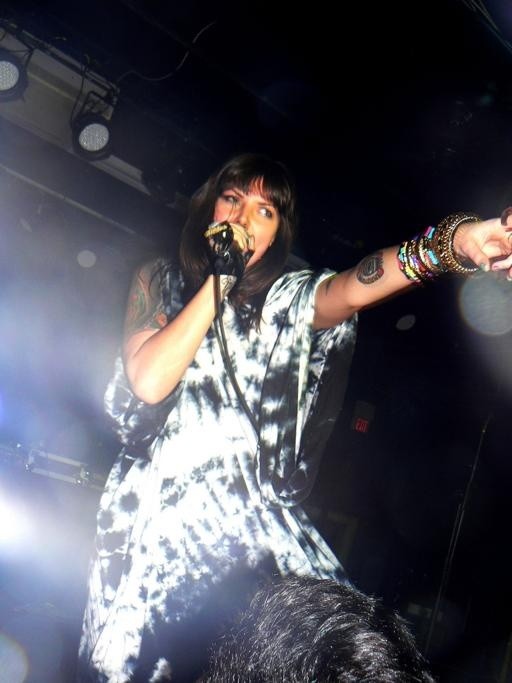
[{"xmin": 210, "ymin": 240, "xmax": 242, "ymax": 276}]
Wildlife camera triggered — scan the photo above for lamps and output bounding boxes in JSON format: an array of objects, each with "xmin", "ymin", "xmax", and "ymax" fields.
[
  {"xmin": 1, "ymin": 48, "xmax": 28, "ymax": 103},
  {"xmin": 71, "ymin": 110, "xmax": 114, "ymax": 162}
]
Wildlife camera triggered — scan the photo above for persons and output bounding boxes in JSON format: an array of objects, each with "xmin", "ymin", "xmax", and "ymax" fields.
[
  {"xmin": 202, "ymin": 573, "xmax": 438, "ymax": 682},
  {"xmin": 73, "ymin": 152, "xmax": 512, "ymax": 682}
]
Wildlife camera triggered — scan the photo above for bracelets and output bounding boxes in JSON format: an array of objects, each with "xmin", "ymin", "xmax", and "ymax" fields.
[{"xmin": 396, "ymin": 209, "xmax": 479, "ymax": 288}]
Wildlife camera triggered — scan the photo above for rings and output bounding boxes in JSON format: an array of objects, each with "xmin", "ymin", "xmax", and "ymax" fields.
[{"xmin": 506, "ymin": 232, "xmax": 512, "ymax": 244}]
